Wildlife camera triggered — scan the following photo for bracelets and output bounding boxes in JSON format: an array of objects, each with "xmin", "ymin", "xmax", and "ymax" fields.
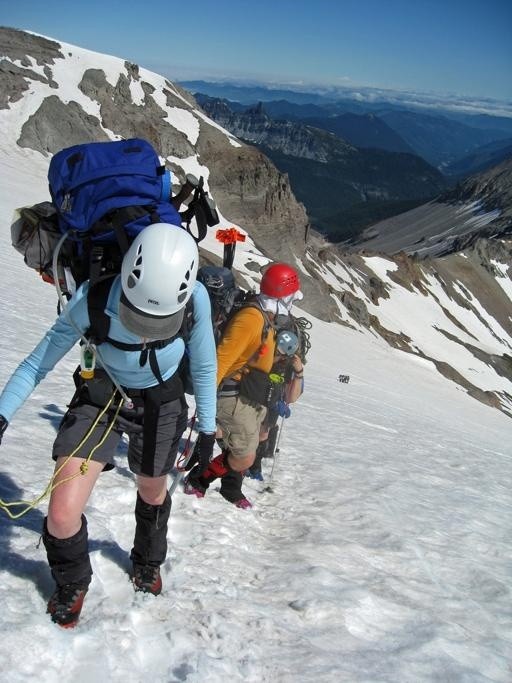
[{"xmin": 295, "ymin": 367, "xmax": 303, "ymax": 373}]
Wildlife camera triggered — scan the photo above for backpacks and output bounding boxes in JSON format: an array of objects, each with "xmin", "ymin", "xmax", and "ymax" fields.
[
  {"xmin": 184, "ymin": 266, "xmax": 271, "ymax": 396},
  {"xmin": 45, "ymin": 137, "xmax": 195, "ymax": 389}
]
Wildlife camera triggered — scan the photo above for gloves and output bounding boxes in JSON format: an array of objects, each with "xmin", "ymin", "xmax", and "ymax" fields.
[{"xmin": 185, "ymin": 429, "xmax": 216, "ymax": 475}]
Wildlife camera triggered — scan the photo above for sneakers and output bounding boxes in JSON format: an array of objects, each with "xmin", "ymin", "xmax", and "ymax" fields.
[
  {"xmin": 47, "ymin": 583, "xmax": 87, "ymax": 627},
  {"xmin": 131, "ymin": 561, "xmax": 162, "ymax": 596},
  {"xmin": 232, "ymin": 495, "xmax": 252, "ymax": 510},
  {"xmin": 184, "ymin": 475, "xmax": 205, "ymax": 499}
]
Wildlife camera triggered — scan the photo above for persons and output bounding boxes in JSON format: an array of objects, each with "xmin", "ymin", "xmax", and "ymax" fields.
[
  {"xmin": 0, "ymin": 223, "xmax": 218, "ymax": 628},
  {"xmin": 215, "ymin": 331, "xmax": 304, "ymax": 481},
  {"xmin": 185, "ymin": 264, "xmax": 299, "ymax": 509}
]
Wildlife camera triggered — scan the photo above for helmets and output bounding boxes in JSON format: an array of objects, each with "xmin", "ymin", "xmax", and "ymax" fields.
[
  {"xmin": 120, "ymin": 221, "xmax": 200, "ymax": 317},
  {"xmin": 276, "ymin": 330, "xmax": 300, "ymax": 357},
  {"xmin": 260, "ymin": 263, "xmax": 299, "ymax": 297}
]
